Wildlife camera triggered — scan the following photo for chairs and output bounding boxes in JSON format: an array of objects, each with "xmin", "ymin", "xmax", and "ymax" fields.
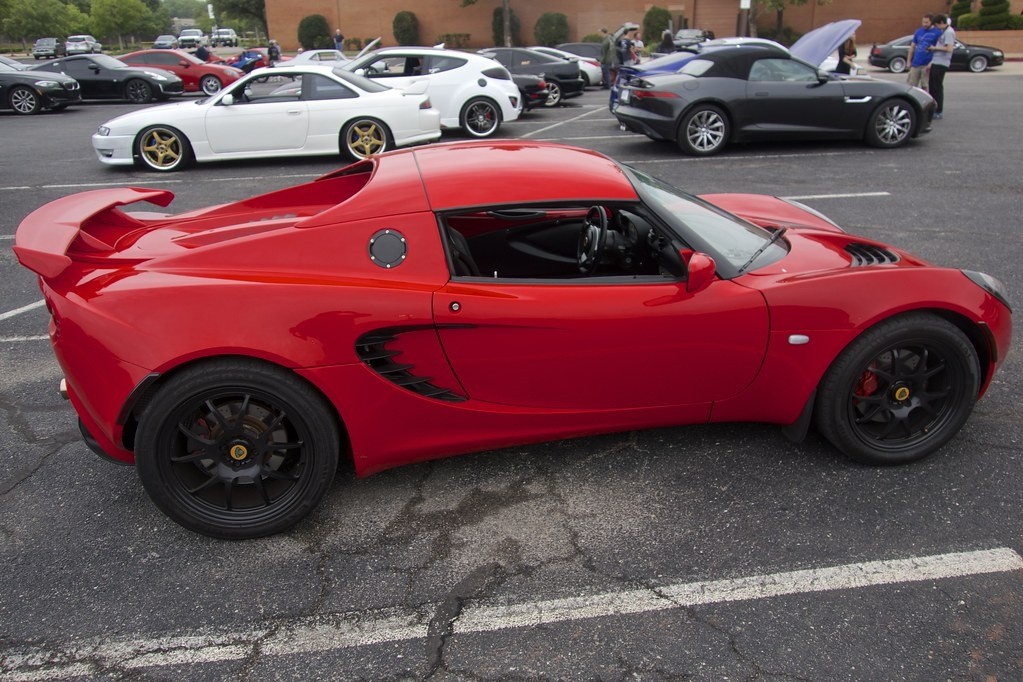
[{"xmin": 446, "ymin": 223, "xmax": 483, "ymax": 277}]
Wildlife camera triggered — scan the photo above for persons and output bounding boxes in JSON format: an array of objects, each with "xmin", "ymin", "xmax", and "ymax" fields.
[
  {"xmin": 267, "ymin": 41, "xmax": 282, "ymax": 66},
  {"xmin": 334, "ymin": 29, "xmax": 344, "ymax": 51},
  {"xmin": 600, "ymin": 27, "xmax": 613, "ymax": 90},
  {"xmin": 172, "ymin": 43, "xmax": 180, "ymax": 50},
  {"xmin": 657, "ymin": 33, "xmax": 674, "ymax": 54},
  {"xmin": 194, "ymin": 43, "xmax": 208, "ymax": 61},
  {"xmin": 621, "ymin": 29, "xmax": 644, "ymax": 65},
  {"xmin": 836, "ymin": 33, "xmax": 858, "ymax": 75},
  {"xmin": 906, "ymin": 15, "xmax": 955, "ymax": 118}
]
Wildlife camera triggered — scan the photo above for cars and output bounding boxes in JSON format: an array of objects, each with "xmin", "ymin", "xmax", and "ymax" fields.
[
  {"xmin": 672, "ymin": 28, "xmax": 707, "ymax": 48},
  {"xmin": 423, "ymin": 42, "xmax": 606, "ymax": 112},
  {"xmin": 152, "ymin": 34, "xmax": 179, "ymax": 50},
  {"xmin": 0, "ymin": 55, "xmax": 83, "ymax": 115},
  {"xmin": 270, "ymin": 41, "xmax": 524, "ymax": 138}
]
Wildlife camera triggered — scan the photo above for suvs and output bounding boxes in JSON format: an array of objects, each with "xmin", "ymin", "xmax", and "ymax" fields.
[
  {"xmin": 179, "ymin": 29, "xmax": 204, "ymax": 49},
  {"xmin": 210, "ymin": 28, "xmax": 238, "ymax": 47},
  {"xmin": 32, "ymin": 37, "xmax": 64, "ymax": 60},
  {"xmin": 65, "ymin": 34, "xmax": 103, "ymax": 57}
]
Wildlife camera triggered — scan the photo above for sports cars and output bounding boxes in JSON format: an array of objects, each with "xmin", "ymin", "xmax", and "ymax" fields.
[
  {"xmin": 111, "ymin": 49, "xmax": 247, "ymax": 98},
  {"xmin": 11, "ymin": 138, "xmax": 1018, "ymax": 542},
  {"xmin": 868, "ymin": 34, "xmax": 1005, "ymax": 74},
  {"xmin": 609, "ymin": 16, "xmax": 940, "ymax": 158},
  {"xmin": 26, "ymin": 53, "xmax": 186, "ymax": 105},
  {"xmin": 91, "ymin": 60, "xmax": 442, "ymax": 174},
  {"xmin": 185, "ymin": 46, "xmax": 390, "ymax": 84}
]
